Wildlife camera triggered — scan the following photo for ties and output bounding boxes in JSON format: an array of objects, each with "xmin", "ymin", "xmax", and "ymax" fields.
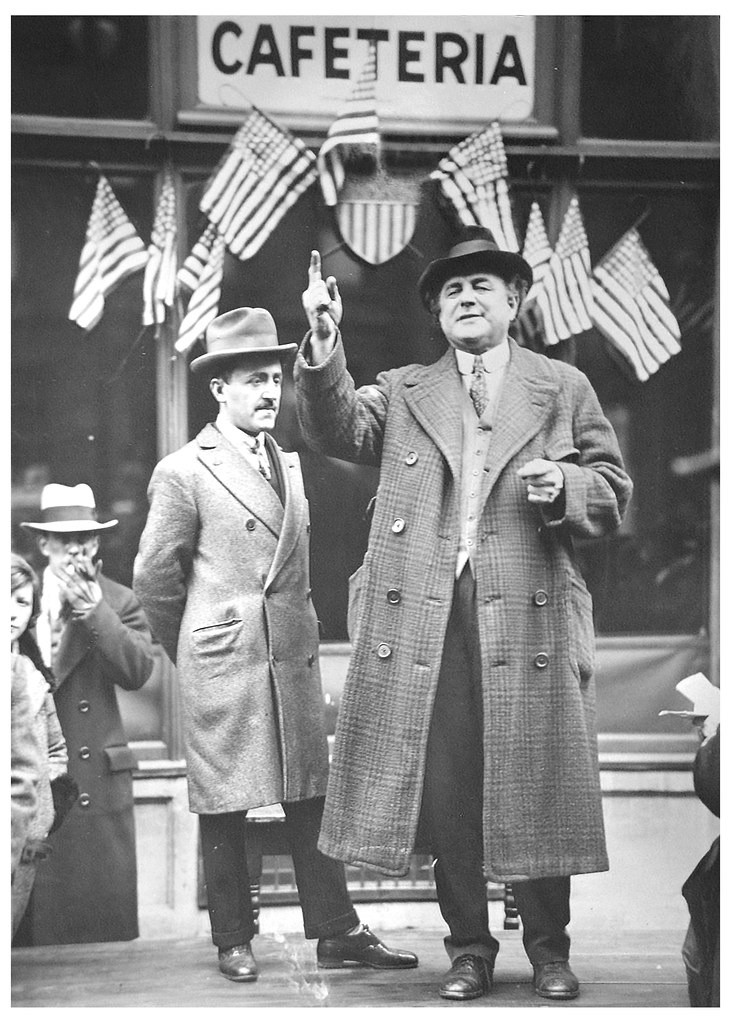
[
  {"xmin": 469, "ymin": 356, "xmax": 490, "ymax": 417},
  {"xmin": 251, "ymin": 440, "xmax": 271, "ymax": 482}
]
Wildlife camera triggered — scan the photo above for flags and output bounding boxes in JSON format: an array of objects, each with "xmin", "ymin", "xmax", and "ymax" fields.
[
  {"xmin": 436, "ymin": 119, "xmax": 519, "ymax": 254},
  {"xmin": 67, "ymin": 177, "xmax": 148, "ymax": 332},
  {"xmin": 536, "ymin": 197, "xmax": 595, "ymax": 345},
  {"xmin": 315, "ymin": 45, "xmax": 380, "ymax": 206},
  {"xmin": 173, "ymin": 224, "xmax": 224, "ymax": 353},
  {"xmin": 591, "ymin": 226, "xmax": 682, "ymax": 382},
  {"xmin": 197, "ymin": 111, "xmax": 319, "ymax": 262},
  {"xmin": 142, "ymin": 175, "xmax": 178, "ymax": 326},
  {"xmin": 517, "ymin": 202, "xmax": 554, "ymax": 313}
]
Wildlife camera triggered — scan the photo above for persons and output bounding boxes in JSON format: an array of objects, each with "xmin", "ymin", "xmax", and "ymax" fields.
[
  {"xmin": 11, "ymin": 482, "xmax": 153, "ymax": 947},
  {"xmin": 681, "ymin": 686, "xmax": 720, "ymax": 1008},
  {"xmin": 291, "ymin": 226, "xmax": 634, "ymax": 1000},
  {"xmin": 133, "ymin": 308, "xmax": 420, "ymax": 983}
]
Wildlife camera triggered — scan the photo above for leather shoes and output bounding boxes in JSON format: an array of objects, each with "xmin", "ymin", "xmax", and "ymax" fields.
[
  {"xmin": 219, "ymin": 943, "xmax": 258, "ymax": 981},
  {"xmin": 534, "ymin": 961, "xmax": 580, "ymax": 996},
  {"xmin": 316, "ymin": 923, "xmax": 418, "ymax": 969},
  {"xmin": 439, "ymin": 954, "xmax": 492, "ymax": 997}
]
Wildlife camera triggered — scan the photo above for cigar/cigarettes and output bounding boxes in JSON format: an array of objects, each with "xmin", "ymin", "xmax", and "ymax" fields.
[{"xmin": 74, "ymin": 555, "xmax": 84, "ymax": 563}]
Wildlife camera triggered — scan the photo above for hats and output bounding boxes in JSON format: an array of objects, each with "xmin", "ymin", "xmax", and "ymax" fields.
[
  {"xmin": 415, "ymin": 226, "xmax": 534, "ymax": 315},
  {"xmin": 189, "ymin": 307, "xmax": 298, "ymax": 373},
  {"xmin": 19, "ymin": 483, "xmax": 118, "ymax": 539}
]
